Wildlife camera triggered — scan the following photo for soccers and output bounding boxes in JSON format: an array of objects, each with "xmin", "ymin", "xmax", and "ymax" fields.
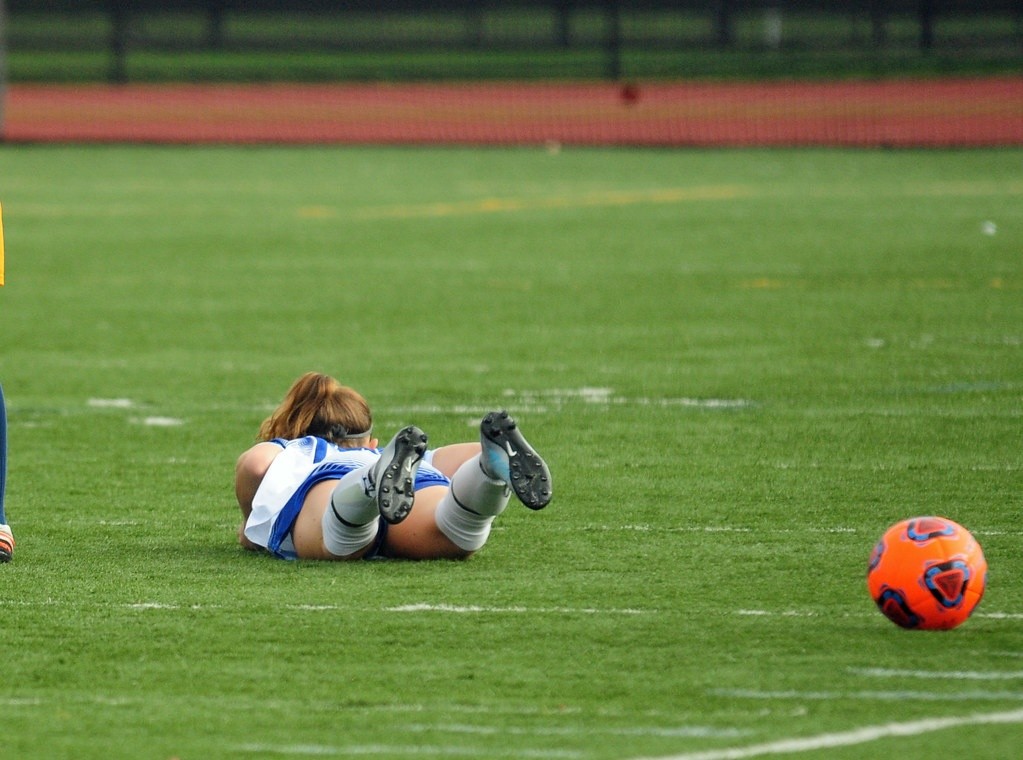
[{"xmin": 868, "ymin": 514, "xmax": 989, "ymax": 632}]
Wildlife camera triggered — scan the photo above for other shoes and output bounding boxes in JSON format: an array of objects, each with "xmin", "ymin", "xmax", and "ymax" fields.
[
  {"xmin": 368, "ymin": 427, "xmax": 428, "ymax": 526},
  {"xmin": 480, "ymin": 411, "xmax": 552, "ymax": 510},
  {"xmin": 0, "ymin": 524, "xmax": 14, "ymax": 563}
]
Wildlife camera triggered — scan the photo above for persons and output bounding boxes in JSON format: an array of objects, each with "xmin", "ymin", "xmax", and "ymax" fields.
[
  {"xmin": 0, "ymin": 197, "xmax": 15, "ymax": 565},
  {"xmin": 235, "ymin": 371, "xmax": 554, "ymax": 563}
]
